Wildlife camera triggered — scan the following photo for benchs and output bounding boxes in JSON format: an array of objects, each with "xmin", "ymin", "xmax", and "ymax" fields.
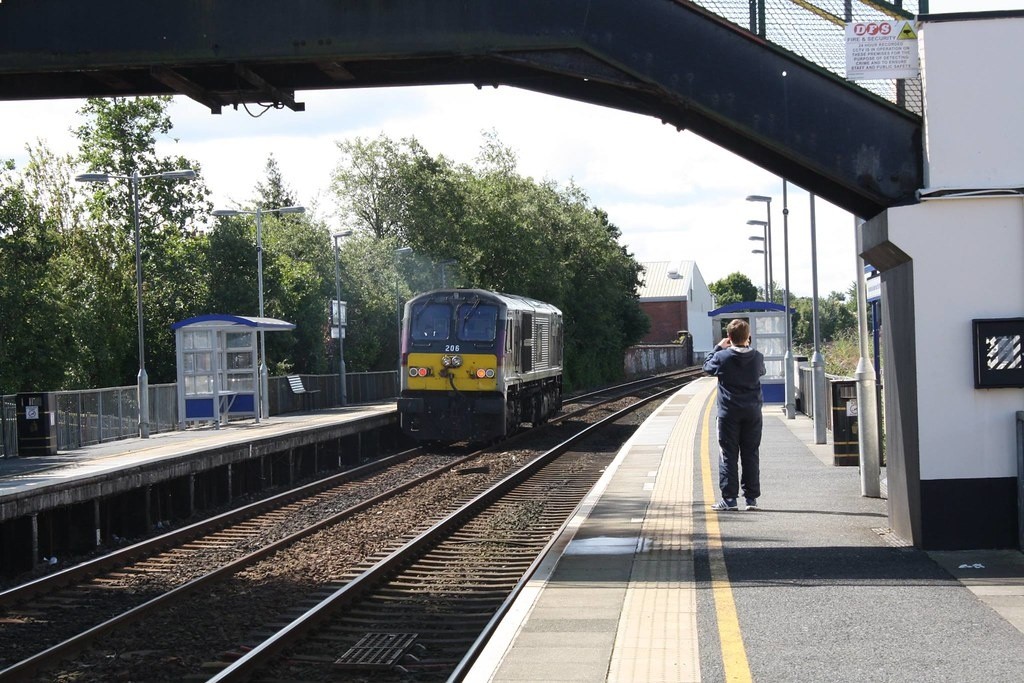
[{"xmin": 286, "ymin": 374, "xmax": 320, "ymax": 412}]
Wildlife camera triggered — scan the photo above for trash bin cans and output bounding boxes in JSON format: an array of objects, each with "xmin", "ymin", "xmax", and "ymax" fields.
[
  {"xmin": 14, "ymin": 391, "xmax": 58, "ymax": 456},
  {"xmin": 829, "ymin": 379, "xmax": 884, "ymax": 468}
]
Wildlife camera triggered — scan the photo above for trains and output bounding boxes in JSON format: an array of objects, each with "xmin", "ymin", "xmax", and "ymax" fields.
[{"xmin": 396, "ymin": 286, "xmax": 564, "ymax": 448}]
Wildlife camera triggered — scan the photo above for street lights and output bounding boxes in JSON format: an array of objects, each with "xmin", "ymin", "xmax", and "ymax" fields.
[
  {"xmin": 747, "ymin": 221, "xmax": 768, "ymax": 302},
  {"xmin": 333, "ymin": 230, "xmax": 352, "ymax": 404},
  {"xmin": 746, "ymin": 196, "xmax": 774, "ymax": 303},
  {"xmin": 211, "ymin": 206, "xmax": 307, "ymax": 419},
  {"xmin": 394, "ymin": 248, "xmax": 412, "ymax": 394},
  {"xmin": 439, "ymin": 260, "xmax": 457, "ymax": 287},
  {"xmin": 76, "ymin": 169, "xmax": 196, "ymax": 438}
]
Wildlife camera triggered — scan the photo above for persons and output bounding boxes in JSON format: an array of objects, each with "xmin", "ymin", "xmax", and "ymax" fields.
[{"xmin": 703, "ymin": 319, "xmax": 766, "ymax": 511}]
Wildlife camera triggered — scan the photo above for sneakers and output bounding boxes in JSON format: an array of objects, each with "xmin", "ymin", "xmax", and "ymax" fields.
[
  {"xmin": 746, "ymin": 499, "xmax": 759, "ymax": 511},
  {"xmin": 711, "ymin": 499, "xmax": 738, "ymax": 510}
]
